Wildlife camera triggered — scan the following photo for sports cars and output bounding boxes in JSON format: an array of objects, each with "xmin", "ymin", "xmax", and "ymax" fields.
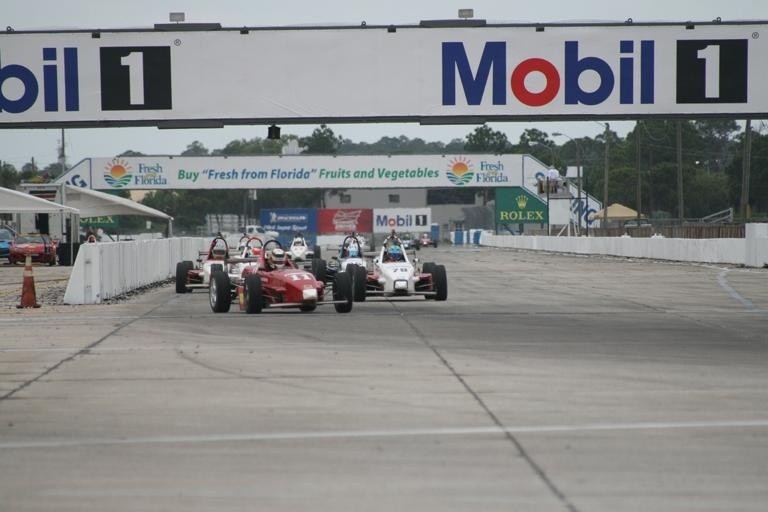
[{"xmin": 175, "ymin": 228, "xmax": 448, "ymax": 313}]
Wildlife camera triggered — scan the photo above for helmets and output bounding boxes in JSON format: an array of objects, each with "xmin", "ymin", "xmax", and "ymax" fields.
[
  {"xmin": 338, "ymin": 246, "xmax": 348, "ymax": 257},
  {"xmin": 212, "ymin": 245, "xmax": 226, "ymax": 258},
  {"xmin": 294, "ymin": 237, "xmax": 302, "ymax": 246},
  {"xmin": 273, "ymin": 248, "xmax": 286, "ymax": 268},
  {"xmin": 387, "ymin": 245, "xmax": 403, "ymax": 261}
]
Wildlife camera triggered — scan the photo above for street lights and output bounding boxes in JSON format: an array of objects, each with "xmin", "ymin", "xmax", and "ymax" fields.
[{"xmin": 529, "ymin": 132, "xmax": 582, "ymax": 236}]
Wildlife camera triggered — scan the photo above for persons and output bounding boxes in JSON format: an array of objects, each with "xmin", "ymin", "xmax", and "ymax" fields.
[
  {"xmin": 87, "ymin": 235, "xmax": 96, "ymax": 243},
  {"xmin": 214, "ymin": 250, "xmax": 225, "ymax": 255}
]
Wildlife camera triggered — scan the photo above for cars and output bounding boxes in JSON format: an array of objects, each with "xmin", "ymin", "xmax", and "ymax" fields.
[{"xmin": 0, "ymin": 228, "xmax": 57, "ymax": 265}]
